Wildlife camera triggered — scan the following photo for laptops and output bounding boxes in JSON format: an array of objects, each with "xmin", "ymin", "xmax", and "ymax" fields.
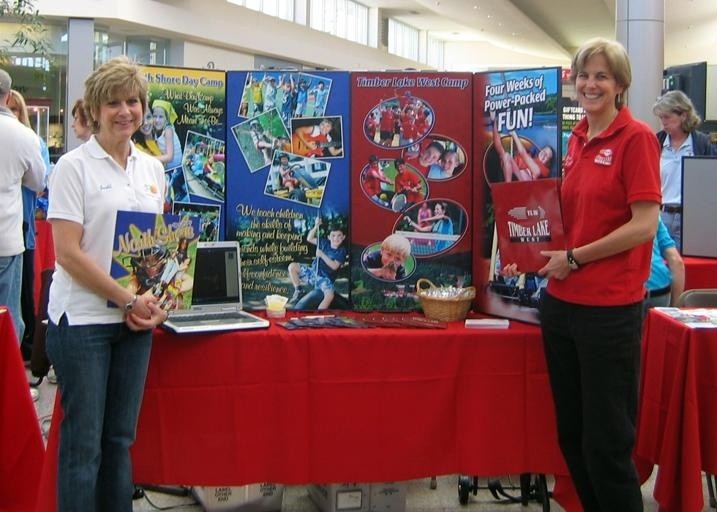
[{"xmin": 156, "ymin": 241, "xmax": 270, "ymax": 336}]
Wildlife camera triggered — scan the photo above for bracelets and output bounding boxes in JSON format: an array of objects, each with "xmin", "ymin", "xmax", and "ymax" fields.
[{"xmin": 564, "ymin": 247, "xmax": 584, "ymax": 272}]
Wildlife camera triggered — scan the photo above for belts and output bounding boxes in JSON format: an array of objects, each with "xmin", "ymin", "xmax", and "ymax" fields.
[
  {"xmin": 651, "ymin": 285, "xmax": 670, "ymax": 297},
  {"xmin": 661, "ymin": 205, "xmax": 682, "ymax": 213}
]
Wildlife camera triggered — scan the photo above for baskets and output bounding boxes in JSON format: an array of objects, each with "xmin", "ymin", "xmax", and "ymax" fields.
[{"xmin": 416, "ymin": 278, "xmax": 476, "ymax": 322}]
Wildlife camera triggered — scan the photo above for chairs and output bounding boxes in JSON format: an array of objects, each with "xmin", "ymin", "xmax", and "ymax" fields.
[{"xmin": 678, "ymin": 288, "xmax": 717, "ymax": 508}]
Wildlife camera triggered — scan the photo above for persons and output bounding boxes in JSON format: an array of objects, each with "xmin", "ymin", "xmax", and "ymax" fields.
[
  {"xmin": 130, "ymin": 73, "xmax": 553, "ymax": 310},
  {"xmin": 44, "ymin": 59, "xmax": 174, "ymax": 512},
  {"xmin": 502, "ymin": 38, "xmax": 659, "ymax": 512},
  {"xmin": 652, "ymin": 88, "xmax": 717, "ymax": 252},
  {"xmin": 5, "ymin": 90, "xmax": 52, "ymax": 367},
  {"xmin": 642, "ymin": 216, "xmax": 686, "ymax": 318},
  {"xmin": 0, "ymin": 68, "xmax": 43, "ymax": 400},
  {"xmin": 71, "ymin": 99, "xmax": 92, "ymax": 140}
]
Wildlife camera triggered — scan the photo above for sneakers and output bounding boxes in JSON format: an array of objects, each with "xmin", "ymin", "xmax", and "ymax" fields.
[{"xmin": 290, "ymin": 288, "xmax": 306, "ymax": 303}]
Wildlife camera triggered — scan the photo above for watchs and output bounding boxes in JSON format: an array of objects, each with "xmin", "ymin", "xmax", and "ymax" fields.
[{"xmin": 124, "ymin": 295, "xmax": 136, "ymax": 315}]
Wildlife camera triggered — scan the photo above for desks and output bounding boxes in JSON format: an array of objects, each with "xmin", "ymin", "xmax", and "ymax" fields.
[
  {"xmin": 37, "ymin": 310, "xmax": 570, "ymax": 512},
  {"xmin": 634, "ymin": 306, "xmax": 717, "ymax": 512},
  {"xmin": 663, "ymin": 256, "xmax": 717, "ymax": 292},
  {"xmin": 0, "ymin": 306, "xmax": 46, "ymax": 512}
]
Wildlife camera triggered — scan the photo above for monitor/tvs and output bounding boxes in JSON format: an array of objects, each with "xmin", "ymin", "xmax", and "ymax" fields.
[{"xmin": 662, "ymin": 61, "xmax": 707, "ymax": 124}]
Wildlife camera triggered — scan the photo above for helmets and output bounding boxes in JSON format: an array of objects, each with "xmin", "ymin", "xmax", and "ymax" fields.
[
  {"xmin": 424, "ymin": 109, "xmax": 430, "ymax": 116},
  {"xmin": 278, "ymin": 153, "xmax": 289, "ymax": 160}
]
